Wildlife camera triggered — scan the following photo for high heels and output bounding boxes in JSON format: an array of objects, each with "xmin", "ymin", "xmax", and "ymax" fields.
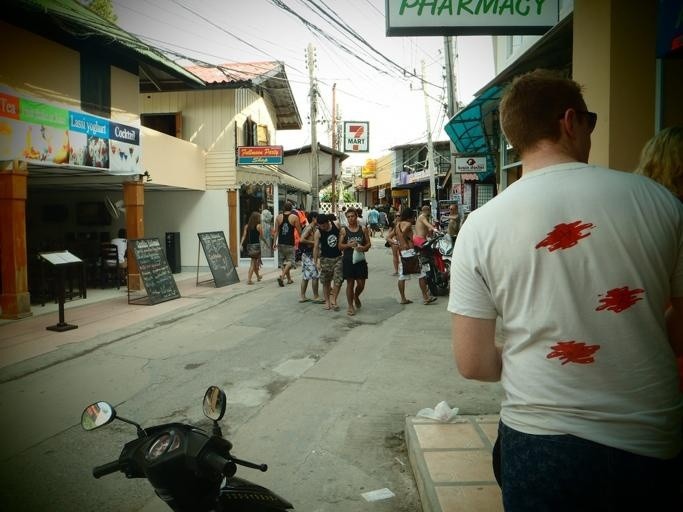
[{"xmin": 257, "ymin": 275, "xmax": 262, "ymax": 281}]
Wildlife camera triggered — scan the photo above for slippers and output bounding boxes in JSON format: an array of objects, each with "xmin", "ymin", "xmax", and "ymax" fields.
[
  {"xmin": 299, "ymin": 299, "xmax": 311, "ymax": 302},
  {"xmin": 310, "ymin": 297, "xmax": 326, "ymax": 302},
  {"xmin": 347, "ymin": 305, "xmax": 355, "ymax": 315},
  {"xmin": 354, "ymin": 294, "xmax": 361, "ymax": 308},
  {"xmin": 287, "ymin": 281, "xmax": 293, "ymax": 284},
  {"xmin": 278, "ymin": 279, "xmax": 284, "ymax": 286}
]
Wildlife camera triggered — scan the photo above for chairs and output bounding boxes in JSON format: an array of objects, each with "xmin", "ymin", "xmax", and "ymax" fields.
[{"xmin": 101, "ymin": 244, "xmax": 128, "ymax": 289}]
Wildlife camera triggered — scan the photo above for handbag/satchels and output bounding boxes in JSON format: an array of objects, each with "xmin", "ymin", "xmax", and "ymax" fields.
[
  {"xmin": 299, "ymin": 243, "xmax": 310, "ymax": 253},
  {"xmin": 385, "ymin": 242, "xmax": 391, "ymax": 247},
  {"xmin": 352, "ymin": 247, "xmax": 366, "ymax": 264},
  {"xmin": 246, "ymin": 246, "xmax": 260, "ymax": 259},
  {"xmin": 401, "ymin": 256, "xmax": 421, "ymax": 275}
]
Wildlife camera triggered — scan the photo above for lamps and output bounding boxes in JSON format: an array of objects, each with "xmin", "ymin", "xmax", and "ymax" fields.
[{"xmin": 139, "ymin": 171, "xmax": 152, "ymax": 182}]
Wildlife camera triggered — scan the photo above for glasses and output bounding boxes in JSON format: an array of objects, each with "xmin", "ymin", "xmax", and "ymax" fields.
[
  {"xmin": 395, "ymin": 215, "xmax": 401, "ymax": 217},
  {"xmin": 560, "ymin": 110, "xmax": 597, "ymax": 132}
]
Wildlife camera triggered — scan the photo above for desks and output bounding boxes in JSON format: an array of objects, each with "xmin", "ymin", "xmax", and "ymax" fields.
[{"xmin": 41, "ymin": 258, "xmax": 86, "ymax": 306}]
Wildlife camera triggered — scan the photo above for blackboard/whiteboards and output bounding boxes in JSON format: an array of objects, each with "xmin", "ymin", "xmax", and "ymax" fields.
[
  {"xmin": 126, "ymin": 237, "xmax": 181, "ymax": 306},
  {"xmin": 196, "ymin": 231, "xmax": 240, "ymax": 288}
]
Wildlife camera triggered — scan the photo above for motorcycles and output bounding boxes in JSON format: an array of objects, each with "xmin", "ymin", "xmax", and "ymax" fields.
[
  {"xmin": 81, "ymin": 385, "xmax": 295, "ymax": 512},
  {"xmin": 417, "ymin": 234, "xmax": 452, "ymax": 298}
]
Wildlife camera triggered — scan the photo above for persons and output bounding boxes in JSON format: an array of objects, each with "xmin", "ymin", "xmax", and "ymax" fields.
[
  {"xmin": 240, "ymin": 211, "xmax": 270, "ymax": 285},
  {"xmin": 630, "ymin": 123, "xmax": 679, "ymax": 196},
  {"xmin": 445, "ymin": 68, "xmax": 681, "ymax": 511},
  {"xmin": 272, "ymin": 202, "xmax": 461, "ymax": 316},
  {"xmin": 104, "ymin": 229, "xmax": 128, "ymax": 285}
]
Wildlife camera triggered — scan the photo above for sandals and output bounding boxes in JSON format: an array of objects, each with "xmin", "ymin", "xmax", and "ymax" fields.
[
  {"xmin": 424, "ymin": 297, "xmax": 437, "ymax": 305},
  {"xmin": 400, "ymin": 299, "xmax": 412, "ymax": 304}
]
